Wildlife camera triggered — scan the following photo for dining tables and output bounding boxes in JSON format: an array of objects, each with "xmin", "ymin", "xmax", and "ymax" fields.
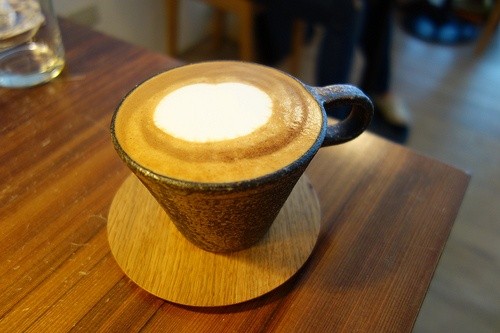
[{"xmin": 2, "ymin": 14, "xmax": 474, "ymax": 332}]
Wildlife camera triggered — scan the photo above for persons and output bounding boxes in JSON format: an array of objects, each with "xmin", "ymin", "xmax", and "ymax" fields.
[{"xmin": 260, "ymin": 0, "xmax": 414, "ymax": 145}]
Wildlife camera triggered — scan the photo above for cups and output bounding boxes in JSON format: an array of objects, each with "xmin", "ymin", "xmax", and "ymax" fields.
[
  {"xmin": 111, "ymin": 61, "xmax": 376, "ymax": 254},
  {"xmin": 0, "ymin": 0, "xmax": 64, "ymax": 89}
]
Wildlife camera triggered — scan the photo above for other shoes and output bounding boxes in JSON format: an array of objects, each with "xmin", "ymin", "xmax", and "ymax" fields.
[{"xmin": 368, "ymin": 96, "xmax": 408, "ymax": 146}]
